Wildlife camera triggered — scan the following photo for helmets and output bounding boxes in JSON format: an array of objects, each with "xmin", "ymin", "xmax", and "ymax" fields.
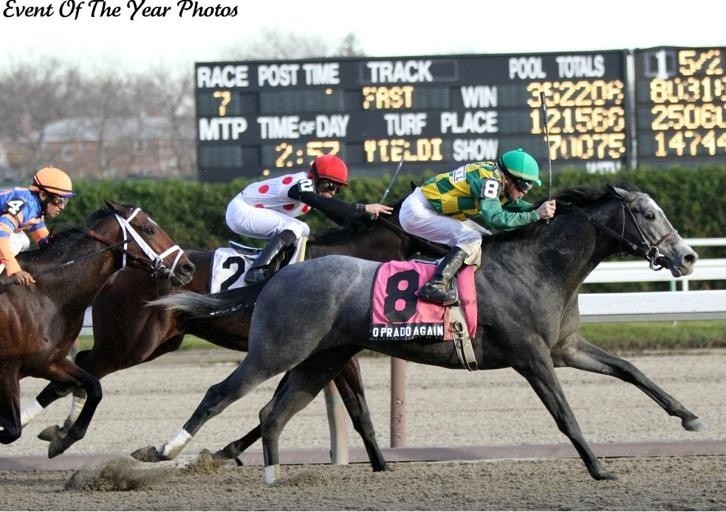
[
  {"xmin": 29, "ymin": 165, "xmax": 77, "ymax": 197},
  {"xmin": 308, "ymin": 155, "xmax": 349, "ymax": 186},
  {"xmin": 499, "ymin": 148, "xmax": 542, "ymax": 187}
]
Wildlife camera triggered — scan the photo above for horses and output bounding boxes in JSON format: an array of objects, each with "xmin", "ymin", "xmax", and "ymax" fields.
[
  {"xmin": 0, "ymin": 199, "xmax": 195, "ymax": 459},
  {"xmin": 130, "ymin": 180, "xmax": 705, "ymax": 485},
  {"xmin": 20, "ymin": 181, "xmax": 450, "ymax": 471}
]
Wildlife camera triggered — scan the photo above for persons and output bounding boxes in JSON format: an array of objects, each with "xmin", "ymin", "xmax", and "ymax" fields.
[
  {"xmin": 399, "ymin": 147, "xmax": 557, "ymax": 307},
  {"xmin": 224, "ymin": 154, "xmax": 394, "ymax": 285},
  {"xmin": 0, "ymin": 165, "xmax": 74, "ymax": 288}
]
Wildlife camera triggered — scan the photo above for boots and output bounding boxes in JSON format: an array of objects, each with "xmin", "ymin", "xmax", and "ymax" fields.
[
  {"xmin": 417, "ymin": 246, "xmax": 469, "ymax": 306},
  {"xmin": 244, "ymin": 237, "xmax": 286, "ymax": 284}
]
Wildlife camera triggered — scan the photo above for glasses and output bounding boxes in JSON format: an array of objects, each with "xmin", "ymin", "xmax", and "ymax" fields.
[
  {"xmin": 312, "ymin": 161, "xmax": 340, "ymax": 193},
  {"xmin": 35, "ymin": 173, "xmax": 69, "ymax": 206},
  {"xmin": 501, "ymin": 155, "xmax": 534, "ymax": 192}
]
